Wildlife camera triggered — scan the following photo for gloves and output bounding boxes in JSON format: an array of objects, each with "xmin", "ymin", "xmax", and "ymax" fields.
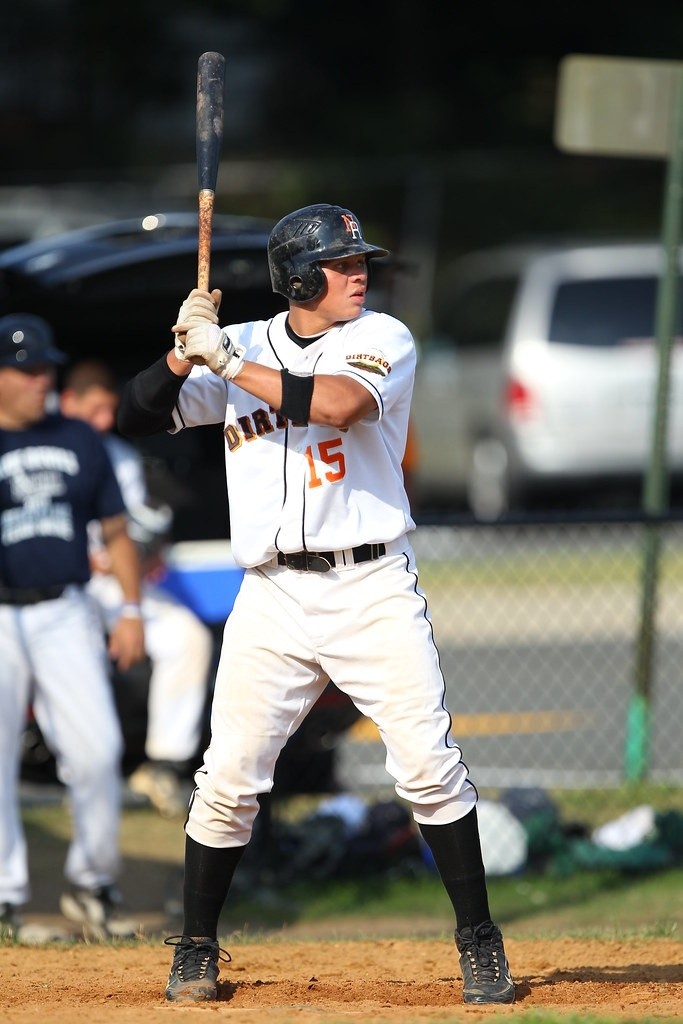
[
  {"xmin": 175, "ymin": 288, "xmax": 222, "ymax": 363},
  {"xmin": 172, "ymin": 321, "xmax": 247, "ymax": 380}
]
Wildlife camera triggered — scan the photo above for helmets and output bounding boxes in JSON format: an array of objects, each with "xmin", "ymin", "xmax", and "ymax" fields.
[{"xmin": 269, "ymin": 204, "xmax": 389, "ymax": 302}]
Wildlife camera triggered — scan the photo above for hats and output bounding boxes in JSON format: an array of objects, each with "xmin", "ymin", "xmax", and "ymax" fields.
[{"xmin": 1, "ymin": 313, "xmax": 67, "ymax": 367}]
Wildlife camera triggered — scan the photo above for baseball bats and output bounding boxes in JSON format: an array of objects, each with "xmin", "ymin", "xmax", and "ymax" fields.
[{"xmin": 184, "ymin": 50, "xmax": 228, "ymax": 366}]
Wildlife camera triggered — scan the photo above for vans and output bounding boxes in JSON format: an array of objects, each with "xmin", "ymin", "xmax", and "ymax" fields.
[
  {"xmin": 1, "ymin": 205, "xmax": 401, "ymax": 776},
  {"xmin": 401, "ymin": 238, "xmax": 683, "ymax": 523}
]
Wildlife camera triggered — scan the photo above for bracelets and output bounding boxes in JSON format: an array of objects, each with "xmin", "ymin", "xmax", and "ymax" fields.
[{"xmin": 119, "ymin": 600, "xmax": 144, "ymax": 620}]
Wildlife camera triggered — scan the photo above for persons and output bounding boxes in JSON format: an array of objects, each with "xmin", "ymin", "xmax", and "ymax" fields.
[
  {"xmin": 41, "ymin": 362, "xmax": 215, "ymax": 818},
  {"xmin": 130, "ymin": 207, "xmax": 528, "ymax": 1009},
  {"xmin": 0, "ymin": 324, "xmax": 141, "ymax": 941}
]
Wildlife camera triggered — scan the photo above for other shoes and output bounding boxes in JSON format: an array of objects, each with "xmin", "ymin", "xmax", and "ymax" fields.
[{"xmin": 130, "ymin": 766, "xmax": 190, "ymax": 817}]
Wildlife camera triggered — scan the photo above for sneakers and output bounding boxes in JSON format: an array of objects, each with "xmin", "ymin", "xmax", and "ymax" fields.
[
  {"xmin": 0, "ymin": 903, "xmax": 76, "ymax": 948},
  {"xmin": 166, "ymin": 936, "xmax": 219, "ymax": 1001},
  {"xmin": 61, "ymin": 884, "xmax": 142, "ymax": 942},
  {"xmin": 455, "ymin": 925, "xmax": 516, "ymax": 1003}
]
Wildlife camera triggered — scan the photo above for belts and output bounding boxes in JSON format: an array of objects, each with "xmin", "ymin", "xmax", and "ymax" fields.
[{"xmin": 265, "ymin": 543, "xmax": 386, "ymax": 572}]
